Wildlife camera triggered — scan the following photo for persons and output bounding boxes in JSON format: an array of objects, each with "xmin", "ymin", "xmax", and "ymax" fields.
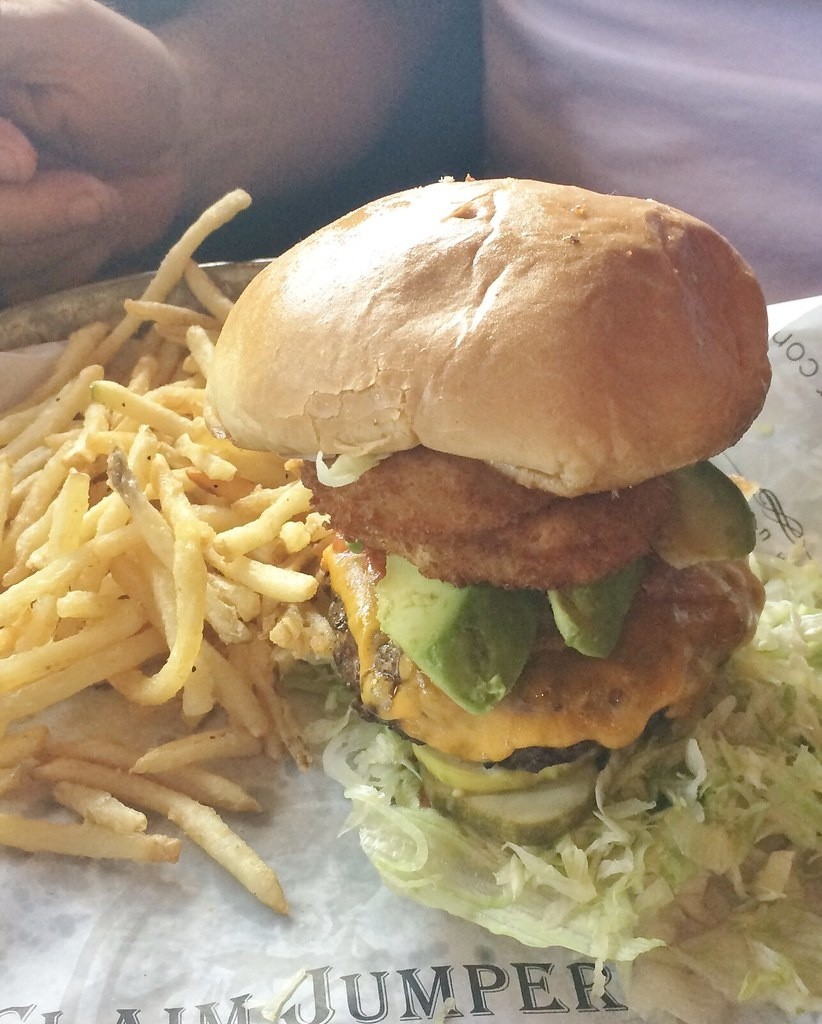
[{"xmin": 0, "ymin": 0, "xmax": 822, "ymax": 308}]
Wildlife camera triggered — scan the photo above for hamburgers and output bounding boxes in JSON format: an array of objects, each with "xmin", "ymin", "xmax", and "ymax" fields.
[{"xmin": 207, "ymin": 176, "xmax": 822, "ymax": 1024}]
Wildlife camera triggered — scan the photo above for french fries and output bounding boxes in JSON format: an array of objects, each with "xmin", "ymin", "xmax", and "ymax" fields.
[{"xmin": 0, "ymin": 188, "xmax": 346, "ymax": 918}]
